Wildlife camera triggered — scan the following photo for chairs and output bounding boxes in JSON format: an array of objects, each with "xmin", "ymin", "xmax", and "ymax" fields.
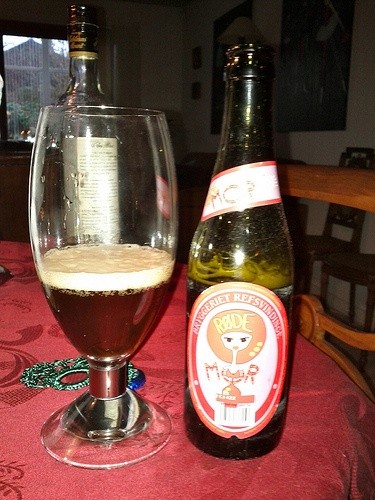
[
  {"xmin": 276, "ymin": 164, "xmax": 375, "ymax": 401},
  {"xmin": 321, "ymin": 253, "xmax": 375, "ymax": 365},
  {"xmin": 290, "ymin": 148, "xmax": 375, "ymax": 320}
]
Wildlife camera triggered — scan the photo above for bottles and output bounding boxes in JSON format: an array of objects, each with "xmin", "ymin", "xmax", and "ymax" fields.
[
  {"xmin": 184, "ymin": 43, "xmax": 295, "ymax": 462},
  {"xmin": 42, "ymin": 5, "xmax": 131, "ymax": 245}
]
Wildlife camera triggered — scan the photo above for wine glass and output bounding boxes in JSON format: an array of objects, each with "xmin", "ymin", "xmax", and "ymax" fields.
[{"xmin": 29, "ymin": 105, "xmax": 177, "ymax": 470}]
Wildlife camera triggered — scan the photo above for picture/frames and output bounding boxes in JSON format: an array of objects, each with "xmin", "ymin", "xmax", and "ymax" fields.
[
  {"xmin": 211, "ymin": 0, "xmax": 252, "ymax": 135},
  {"xmin": 275, "ymin": 1, "xmax": 355, "ymax": 135}
]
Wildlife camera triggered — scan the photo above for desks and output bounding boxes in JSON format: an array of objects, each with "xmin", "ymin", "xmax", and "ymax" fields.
[{"xmin": 1, "ymin": 240, "xmax": 375, "ymax": 499}]
